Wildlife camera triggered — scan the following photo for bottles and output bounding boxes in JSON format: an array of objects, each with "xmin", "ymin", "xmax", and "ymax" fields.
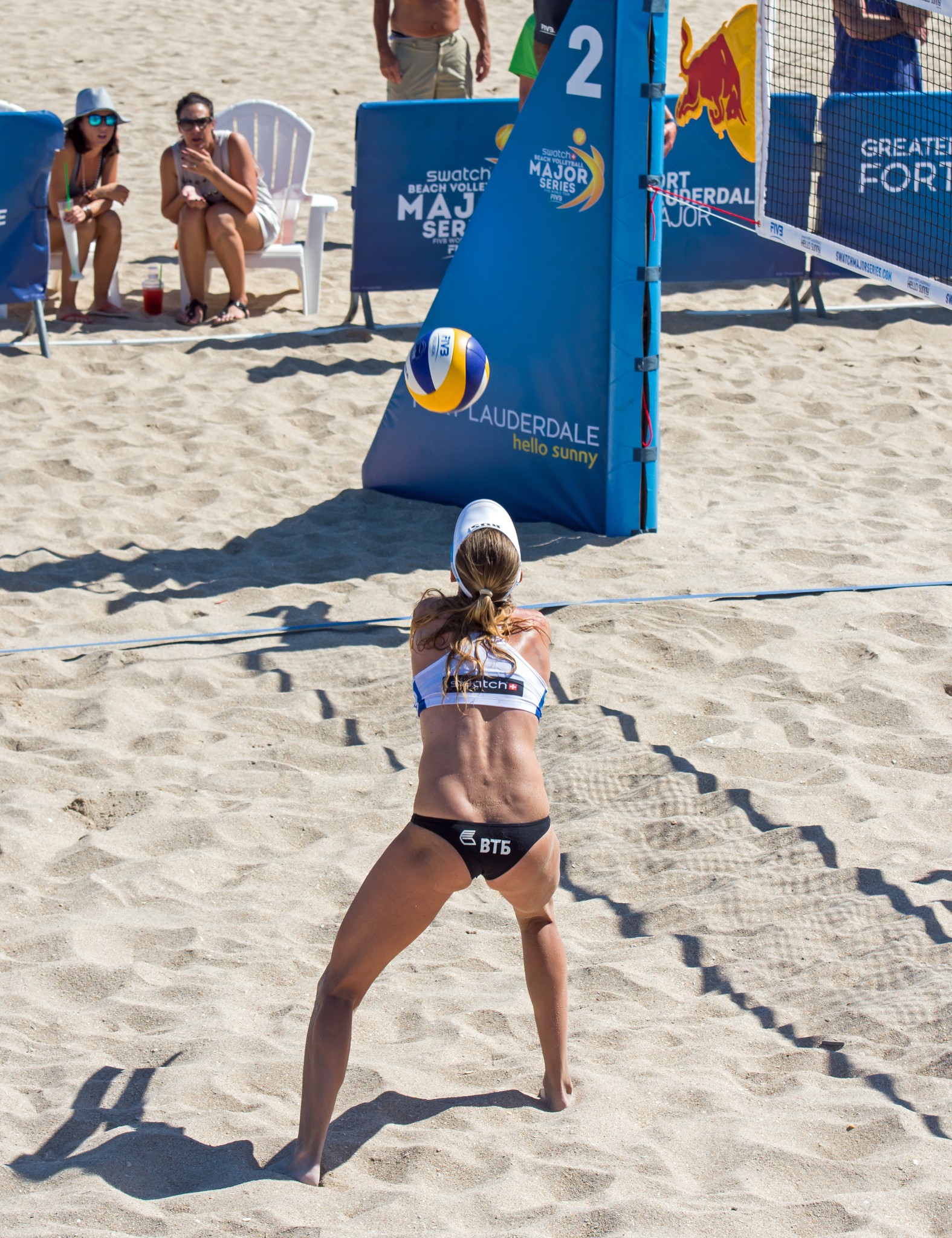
[{"xmin": 142, "ymin": 266, "xmax": 164, "ymax": 316}]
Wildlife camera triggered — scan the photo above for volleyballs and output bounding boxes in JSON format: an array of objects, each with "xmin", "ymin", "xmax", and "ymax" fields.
[{"xmin": 404, "ymin": 327, "xmax": 490, "ymax": 414}]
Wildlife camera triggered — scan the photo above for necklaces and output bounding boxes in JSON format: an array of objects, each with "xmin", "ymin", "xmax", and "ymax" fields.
[{"xmin": 81, "ymin": 153, "xmax": 102, "ymax": 194}]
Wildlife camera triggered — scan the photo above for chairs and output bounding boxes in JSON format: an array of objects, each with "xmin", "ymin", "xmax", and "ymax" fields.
[
  {"xmin": 174, "ymin": 100, "xmax": 339, "ymax": 317},
  {"xmin": 0, "ymin": 97, "xmax": 130, "ymax": 316}
]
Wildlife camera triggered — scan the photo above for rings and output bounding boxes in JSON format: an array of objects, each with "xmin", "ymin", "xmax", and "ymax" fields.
[{"xmin": 193, "ymin": 164, "xmax": 197, "ymax": 169}]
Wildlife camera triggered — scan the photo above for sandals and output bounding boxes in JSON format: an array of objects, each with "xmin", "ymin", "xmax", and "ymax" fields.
[
  {"xmin": 175, "ymin": 298, "xmax": 208, "ymax": 327},
  {"xmin": 211, "ymin": 298, "xmax": 250, "ymax": 327}
]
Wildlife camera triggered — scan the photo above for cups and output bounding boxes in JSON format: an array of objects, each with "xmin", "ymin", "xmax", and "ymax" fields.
[{"xmin": 142, "ymin": 283, "xmax": 164, "ymax": 316}]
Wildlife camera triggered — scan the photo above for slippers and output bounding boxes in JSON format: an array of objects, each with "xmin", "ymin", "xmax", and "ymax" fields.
[
  {"xmin": 87, "ymin": 302, "xmax": 130, "ymax": 318},
  {"xmin": 52, "ymin": 310, "xmax": 93, "ymax": 326}
]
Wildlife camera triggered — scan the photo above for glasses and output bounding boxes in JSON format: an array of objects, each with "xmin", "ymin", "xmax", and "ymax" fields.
[
  {"xmin": 84, "ymin": 114, "xmax": 118, "ymax": 126},
  {"xmin": 177, "ymin": 116, "xmax": 213, "ymax": 131}
]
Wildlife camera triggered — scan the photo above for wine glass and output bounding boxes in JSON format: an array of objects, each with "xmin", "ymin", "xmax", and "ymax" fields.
[{"xmin": 57, "ymin": 199, "xmax": 84, "ymax": 281}]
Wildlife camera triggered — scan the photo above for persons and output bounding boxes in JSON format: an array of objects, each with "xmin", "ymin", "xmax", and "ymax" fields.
[
  {"xmin": 508, "ymin": 14, "xmax": 539, "ymax": 113},
  {"xmin": 263, "ymin": 500, "xmax": 572, "ymax": 1186},
  {"xmin": 159, "ymin": 92, "xmax": 281, "ymax": 327},
  {"xmin": 47, "ymin": 85, "xmax": 132, "ymax": 325},
  {"xmin": 373, "ymin": 0, "xmax": 492, "ymax": 102},
  {"xmin": 829, "ymin": 0, "xmax": 930, "ymax": 94},
  {"xmin": 533, "ymin": 0, "xmax": 679, "ymax": 158}
]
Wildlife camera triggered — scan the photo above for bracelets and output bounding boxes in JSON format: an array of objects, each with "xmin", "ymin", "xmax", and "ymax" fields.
[
  {"xmin": 85, "ymin": 190, "xmax": 97, "ymax": 202},
  {"xmin": 85, "ymin": 205, "xmax": 93, "ymax": 218},
  {"xmin": 664, "ymin": 118, "xmax": 678, "ymax": 127}
]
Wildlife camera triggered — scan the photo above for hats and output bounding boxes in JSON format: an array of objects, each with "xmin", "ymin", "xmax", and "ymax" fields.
[
  {"xmin": 451, "ymin": 499, "xmax": 523, "ymax": 602},
  {"xmin": 61, "ymin": 87, "xmax": 132, "ymax": 125}
]
